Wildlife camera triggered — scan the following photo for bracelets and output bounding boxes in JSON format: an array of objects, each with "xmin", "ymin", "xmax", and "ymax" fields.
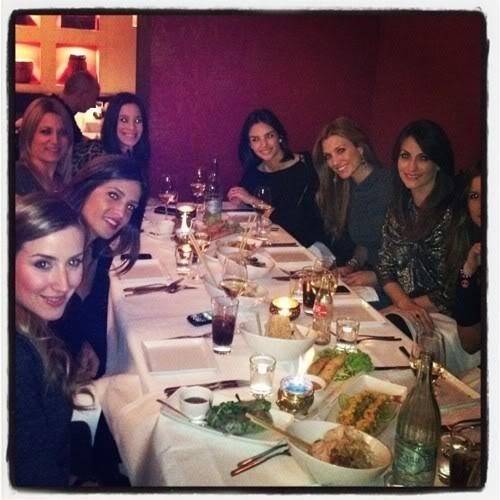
[
  {"xmin": 459, "ymin": 267, "xmax": 472, "ymax": 285},
  {"xmin": 347, "ymin": 256, "xmax": 360, "ymax": 270},
  {"xmin": 252, "ymin": 196, "xmax": 264, "ymax": 210}
]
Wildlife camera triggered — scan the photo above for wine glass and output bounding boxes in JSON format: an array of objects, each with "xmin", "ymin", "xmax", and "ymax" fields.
[
  {"xmin": 411, "ymin": 333, "xmax": 447, "ymax": 393},
  {"xmin": 187, "ymin": 217, "xmax": 213, "ymax": 273},
  {"xmin": 158, "ymin": 175, "xmax": 178, "ymax": 218},
  {"xmin": 221, "ymin": 258, "xmax": 248, "ymax": 299},
  {"xmin": 191, "ymin": 167, "xmax": 205, "ymax": 207},
  {"xmin": 252, "ymin": 184, "xmax": 272, "ymax": 240},
  {"xmin": 248, "ymin": 353, "xmax": 277, "ymax": 401}
]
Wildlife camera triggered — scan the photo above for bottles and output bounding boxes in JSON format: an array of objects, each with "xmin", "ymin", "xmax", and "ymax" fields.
[
  {"xmin": 311, "ymin": 274, "xmax": 332, "ymax": 346},
  {"xmin": 393, "ymin": 352, "xmax": 442, "ymax": 486},
  {"xmin": 205, "ymin": 158, "xmax": 222, "ymax": 221}
]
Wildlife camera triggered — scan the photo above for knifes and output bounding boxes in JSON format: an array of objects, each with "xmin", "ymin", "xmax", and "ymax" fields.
[
  {"xmin": 165, "ymin": 380, "xmax": 246, "ymax": 394},
  {"xmin": 373, "ymin": 365, "xmax": 411, "ymax": 372},
  {"xmin": 398, "ymin": 346, "xmax": 412, "ymax": 359},
  {"xmin": 265, "ymin": 243, "xmax": 299, "ymax": 248}
]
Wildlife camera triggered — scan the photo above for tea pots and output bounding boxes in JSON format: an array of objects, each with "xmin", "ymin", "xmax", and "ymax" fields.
[{"xmin": 435, "ymin": 418, "xmax": 483, "ymax": 487}]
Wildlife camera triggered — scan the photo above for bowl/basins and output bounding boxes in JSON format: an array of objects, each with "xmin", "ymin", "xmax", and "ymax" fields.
[
  {"xmin": 202, "ymin": 273, "xmax": 268, "ymax": 308},
  {"xmin": 238, "ymin": 320, "xmax": 318, "ymax": 360},
  {"xmin": 286, "ymin": 419, "xmax": 393, "ymax": 486},
  {"xmin": 215, "ymin": 245, "xmax": 276, "ymax": 277}
]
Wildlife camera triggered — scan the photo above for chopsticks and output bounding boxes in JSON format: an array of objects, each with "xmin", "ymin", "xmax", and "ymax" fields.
[{"xmin": 242, "ymin": 411, "xmax": 310, "ymax": 451}]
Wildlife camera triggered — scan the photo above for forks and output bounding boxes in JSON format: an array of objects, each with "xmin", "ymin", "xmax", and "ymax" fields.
[
  {"xmin": 230, "ymin": 441, "xmax": 289, "ymax": 478},
  {"xmin": 354, "ymin": 334, "xmax": 404, "ymax": 343},
  {"xmin": 123, "ymin": 278, "xmax": 194, "ymax": 301}
]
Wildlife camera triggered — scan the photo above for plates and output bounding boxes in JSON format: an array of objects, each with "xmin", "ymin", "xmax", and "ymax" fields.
[
  {"xmin": 144, "ymin": 222, "xmax": 175, "ymax": 239},
  {"xmin": 317, "ymin": 373, "xmax": 405, "ymax": 432},
  {"xmin": 264, "ymin": 247, "xmax": 317, "ymax": 263},
  {"xmin": 228, "ymin": 209, "xmax": 270, "ymax": 230},
  {"xmin": 201, "ymin": 234, "xmax": 263, "ymax": 269},
  {"xmin": 332, "ymin": 300, "xmax": 383, "ymax": 324},
  {"xmin": 140, "ymin": 336, "xmax": 220, "ymax": 372},
  {"xmin": 159, "ymin": 387, "xmax": 297, "ymax": 443},
  {"xmin": 111, "ymin": 259, "xmax": 170, "ymax": 282}
]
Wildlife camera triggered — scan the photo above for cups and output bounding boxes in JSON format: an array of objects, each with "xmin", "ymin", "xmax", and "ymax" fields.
[
  {"xmin": 157, "ymin": 222, "xmax": 174, "ymax": 233},
  {"xmin": 175, "ymin": 245, "xmax": 195, "ymax": 276},
  {"xmin": 210, "ymin": 295, "xmax": 240, "ymax": 355},
  {"xmin": 335, "ymin": 316, "xmax": 360, "ymax": 353},
  {"xmin": 178, "ymin": 386, "xmax": 215, "ymax": 420},
  {"xmin": 290, "ymin": 259, "xmax": 330, "ymax": 313}
]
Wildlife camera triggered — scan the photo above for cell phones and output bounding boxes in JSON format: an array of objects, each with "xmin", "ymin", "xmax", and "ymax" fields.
[
  {"xmin": 336, "ymin": 286, "xmax": 350, "ymax": 294},
  {"xmin": 187, "ymin": 311, "xmax": 212, "ymax": 326},
  {"xmin": 155, "ymin": 205, "xmax": 177, "ymax": 215}
]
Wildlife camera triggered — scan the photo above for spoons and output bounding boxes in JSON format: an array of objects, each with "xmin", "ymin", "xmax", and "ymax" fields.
[{"xmin": 154, "ymin": 397, "xmax": 190, "ymax": 420}]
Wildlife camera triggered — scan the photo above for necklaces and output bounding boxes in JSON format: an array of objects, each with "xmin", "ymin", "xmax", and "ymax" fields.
[{"xmin": 261, "ymin": 162, "xmax": 276, "ymax": 195}]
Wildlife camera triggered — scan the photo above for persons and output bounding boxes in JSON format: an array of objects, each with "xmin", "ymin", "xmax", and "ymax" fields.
[
  {"xmin": 49, "ymin": 71, "xmax": 100, "ymax": 145},
  {"xmin": 16, "ymin": 97, "xmax": 73, "ymax": 196},
  {"xmin": 306, "ymin": 114, "xmax": 399, "ymax": 291},
  {"xmin": 12, "ymin": 192, "xmax": 132, "ymax": 487},
  {"xmin": 61, "ymin": 153, "xmax": 145, "ymax": 369},
  {"xmin": 71, "ymin": 92, "xmax": 145, "ymax": 172},
  {"xmin": 226, "ymin": 110, "xmax": 322, "ymax": 247},
  {"xmin": 421, "ymin": 161, "xmax": 482, "ymax": 393},
  {"xmin": 360, "ymin": 118, "xmax": 473, "ymax": 340}
]
